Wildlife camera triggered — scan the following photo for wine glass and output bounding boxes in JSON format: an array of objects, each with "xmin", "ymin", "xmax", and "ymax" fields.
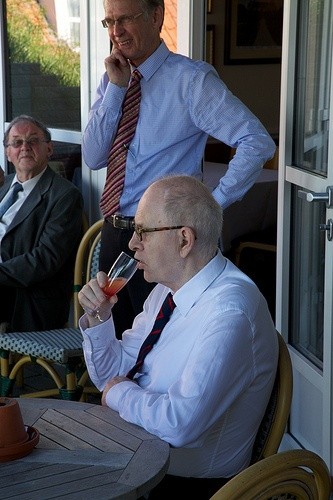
[{"xmin": 84, "ymin": 251, "xmax": 141, "ymax": 324}]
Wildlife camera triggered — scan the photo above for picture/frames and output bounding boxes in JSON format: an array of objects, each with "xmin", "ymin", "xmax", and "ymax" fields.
[{"xmin": 224, "ymin": 0, "xmax": 284, "ymax": 66}]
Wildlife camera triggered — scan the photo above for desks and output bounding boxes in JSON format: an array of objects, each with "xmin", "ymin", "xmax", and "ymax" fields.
[{"xmin": 0, "ymin": 397, "xmax": 170, "ymax": 500}]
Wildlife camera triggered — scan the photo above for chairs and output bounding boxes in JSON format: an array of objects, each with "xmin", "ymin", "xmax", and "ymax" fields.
[{"xmin": 0, "ymin": 217, "xmax": 333, "ymax": 500}]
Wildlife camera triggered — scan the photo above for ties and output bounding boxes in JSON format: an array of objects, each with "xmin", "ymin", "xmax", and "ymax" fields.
[
  {"xmin": 126, "ymin": 292, "xmax": 177, "ymax": 383},
  {"xmin": 100, "ymin": 70, "xmax": 144, "ymax": 219},
  {"xmin": 0, "ymin": 182, "xmax": 23, "ymax": 220}
]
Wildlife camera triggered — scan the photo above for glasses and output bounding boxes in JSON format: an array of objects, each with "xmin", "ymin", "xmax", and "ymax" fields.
[
  {"xmin": 6, "ymin": 137, "xmax": 48, "ymax": 148},
  {"xmin": 101, "ymin": 10, "xmax": 144, "ymax": 28},
  {"xmin": 132, "ymin": 223, "xmax": 198, "ymax": 242}
]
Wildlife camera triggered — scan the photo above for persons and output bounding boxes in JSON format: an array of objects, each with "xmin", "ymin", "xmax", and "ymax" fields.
[
  {"xmin": 81, "ymin": 0, "xmax": 278, "ymax": 340},
  {"xmin": 78, "ymin": 175, "xmax": 279, "ymax": 500},
  {"xmin": 0, "ymin": 115, "xmax": 84, "ymax": 333}
]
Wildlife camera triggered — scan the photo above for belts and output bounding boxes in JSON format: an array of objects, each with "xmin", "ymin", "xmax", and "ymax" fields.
[{"xmin": 106, "ymin": 214, "xmax": 136, "ymax": 229}]
